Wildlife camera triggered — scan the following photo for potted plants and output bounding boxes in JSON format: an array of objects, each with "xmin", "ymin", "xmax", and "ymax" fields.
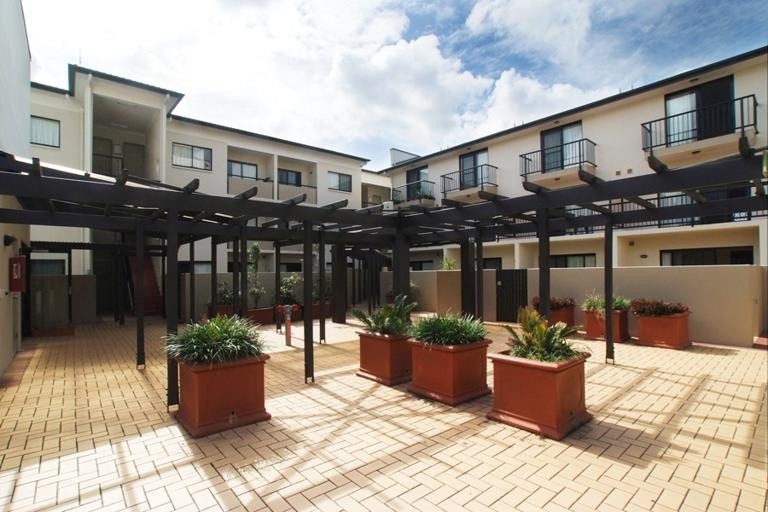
[
  {"xmin": 312, "ymin": 275, "xmax": 332, "ymax": 319},
  {"xmin": 206, "ymin": 281, "xmax": 242, "ymax": 315},
  {"xmin": 387, "ymin": 280, "xmax": 421, "ymax": 303},
  {"xmin": 406, "ymin": 307, "xmax": 493, "ymax": 408},
  {"xmin": 532, "ymin": 296, "xmax": 576, "ymax": 327},
  {"xmin": 352, "ymin": 293, "xmax": 419, "ymax": 387},
  {"xmin": 161, "ymin": 312, "xmax": 272, "ymax": 439},
  {"xmin": 271, "ymin": 271, "xmax": 304, "ymax": 321},
  {"xmin": 580, "ymin": 288, "xmax": 631, "ymax": 343},
  {"xmin": 485, "ymin": 305, "xmax": 594, "ymax": 441},
  {"xmin": 247, "ymin": 286, "xmax": 273, "ymax": 323},
  {"xmin": 629, "ymin": 297, "xmax": 690, "ymax": 350}
]
